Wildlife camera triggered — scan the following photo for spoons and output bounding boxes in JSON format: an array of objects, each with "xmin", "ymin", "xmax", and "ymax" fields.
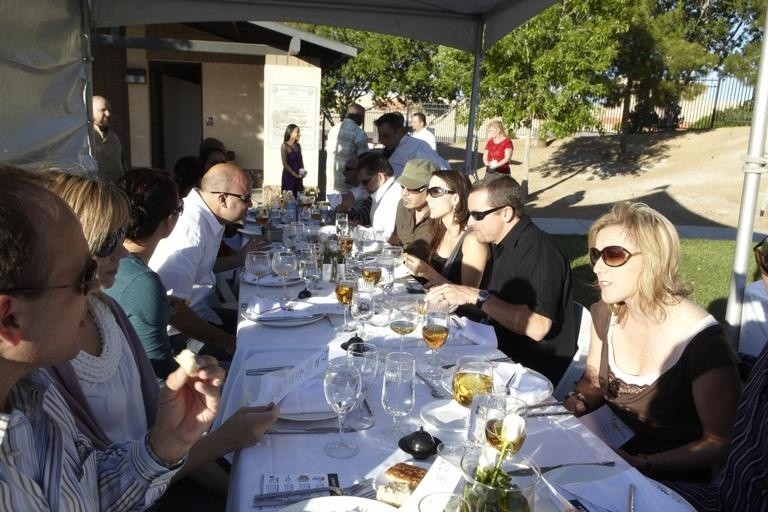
[{"xmin": 414, "ymin": 371, "xmax": 446, "ymax": 399}]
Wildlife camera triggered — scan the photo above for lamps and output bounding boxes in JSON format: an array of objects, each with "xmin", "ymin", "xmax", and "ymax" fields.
[{"xmin": 122, "ymin": 67, "xmax": 147, "ymax": 85}]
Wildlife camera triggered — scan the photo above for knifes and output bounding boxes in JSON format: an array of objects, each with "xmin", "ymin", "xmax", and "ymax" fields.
[
  {"xmin": 264, "ymin": 426, "xmax": 355, "ymax": 435},
  {"xmin": 442, "ymin": 356, "xmax": 512, "ymax": 368},
  {"xmin": 526, "ymin": 411, "xmax": 575, "ymax": 417},
  {"xmin": 508, "ymin": 462, "xmax": 617, "ymax": 474}
]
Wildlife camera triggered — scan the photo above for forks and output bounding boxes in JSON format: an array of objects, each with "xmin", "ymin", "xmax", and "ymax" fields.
[
  {"xmin": 253, "ymin": 489, "xmax": 376, "ymax": 507},
  {"xmin": 255, "ymin": 478, "xmax": 372, "ymax": 496}
]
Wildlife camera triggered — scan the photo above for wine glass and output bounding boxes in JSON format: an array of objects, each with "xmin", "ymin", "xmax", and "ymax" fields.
[
  {"xmin": 441, "ymin": 361, "xmax": 494, "ymax": 438},
  {"xmin": 416, "ymin": 492, "xmax": 473, "ymax": 512},
  {"xmin": 387, "ymin": 295, "xmax": 418, "ymax": 351},
  {"xmin": 485, "ymin": 395, "xmax": 527, "ymax": 474},
  {"xmin": 421, "ymin": 298, "xmax": 449, "ymax": 379},
  {"xmin": 346, "ymin": 344, "xmax": 379, "ymax": 428},
  {"xmin": 382, "ymin": 351, "xmax": 416, "ymax": 442},
  {"xmin": 246, "ymin": 188, "xmax": 406, "ymax": 337},
  {"xmin": 323, "ymin": 364, "xmax": 360, "ymax": 458}
]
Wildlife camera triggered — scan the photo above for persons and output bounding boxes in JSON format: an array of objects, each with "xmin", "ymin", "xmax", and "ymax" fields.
[
  {"xmin": 29, "ymin": 165, "xmax": 281, "ymax": 512},
  {"xmin": 100, "ymin": 169, "xmax": 231, "ymax": 393},
  {"xmin": 655, "ymin": 230, "xmax": 767, "ymax": 511},
  {"xmin": 91, "ymin": 93, "xmax": 126, "ymax": 186},
  {"xmin": 146, "ymin": 102, "xmax": 577, "ymax": 393},
  {"xmin": 559, "ymin": 201, "xmax": 743, "ymax": 483},
  {"xmin": 1, "ymin": 160, "xmax": 226, "ymax": 512}
]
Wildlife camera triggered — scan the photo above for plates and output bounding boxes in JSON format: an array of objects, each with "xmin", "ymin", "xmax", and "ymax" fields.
[
  {"xmin": 376, "ymin": 461, "xmax": 462, "ymax": 508},
  {"xmin": 240, "ymin": 298, "xmax": 327, "ymax": 327},
  {"xmin": 391, "ymin": 260, "xmax": 413, "ymax": 279},
  {"xmin": 526, "ymin": 463, "xmax": 697, "ymax": 512},
  {"xmin": 277, "ymin": 497, "xmax": 401, "ymax": 512},
  {"xmin": 456, "ymin": 361, "xmax": 555, "ymax": 407},
  {"xmin": 242, "ymin": 370, "xmax": 351, "ymax": 422},
  {"xmin": 419, "ymin": 399, "xmax": 469, "ymax": 432},
  {"xmin": 239, "ymin": 270, "xmax": 303, "ymax": 285}
]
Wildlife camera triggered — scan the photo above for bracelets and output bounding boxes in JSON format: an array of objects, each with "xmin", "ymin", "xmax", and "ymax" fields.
[
  {"xmin": 560, "ymin": 391, "xmax": 587, "ymax": 406},
  {"xmin": 142, "ymin": 431, "xmax": 188, "ymax": 470},
  {"xmin": 636, "ymin": 452, "xmax": 652, "ymax": 478}
]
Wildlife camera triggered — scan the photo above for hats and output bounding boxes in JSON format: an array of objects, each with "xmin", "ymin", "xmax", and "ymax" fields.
[{"xmin": 395, "ymin": 159, "xmax": 440, "ymax": 190}]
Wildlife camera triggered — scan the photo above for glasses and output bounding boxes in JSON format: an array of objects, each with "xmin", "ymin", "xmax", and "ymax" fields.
[
  {"xmin": 0, "ymin": 259, "xmax": 97, "ymax": 296},
  {"xmin": 91, "ymin": 222, "xmax": 126, "ymax": 257},
  {"xmin": 401, "ymin": 184, "xmax": 457, "ymax": 198},
  {"xmin": 362, "ymin": 177, "xmax": 372, "ymax": 185},
  {"xmin": 345, "ymin": 166, "xmax": 355, "ymax": 171},
  {"xmin": 173, "ymin": 197, "xmax": 184, "ymax": 215},
  {"xmin": 211, "ymin": 192, "xmax": 252, "ymax": 202},
  {"xmin": 468, "ymin": 205, "xmax": 515, "ymax": 221},
  {"xmin": 590, "ymin": 246, "xmax": 641, "ymax": 267}
]
